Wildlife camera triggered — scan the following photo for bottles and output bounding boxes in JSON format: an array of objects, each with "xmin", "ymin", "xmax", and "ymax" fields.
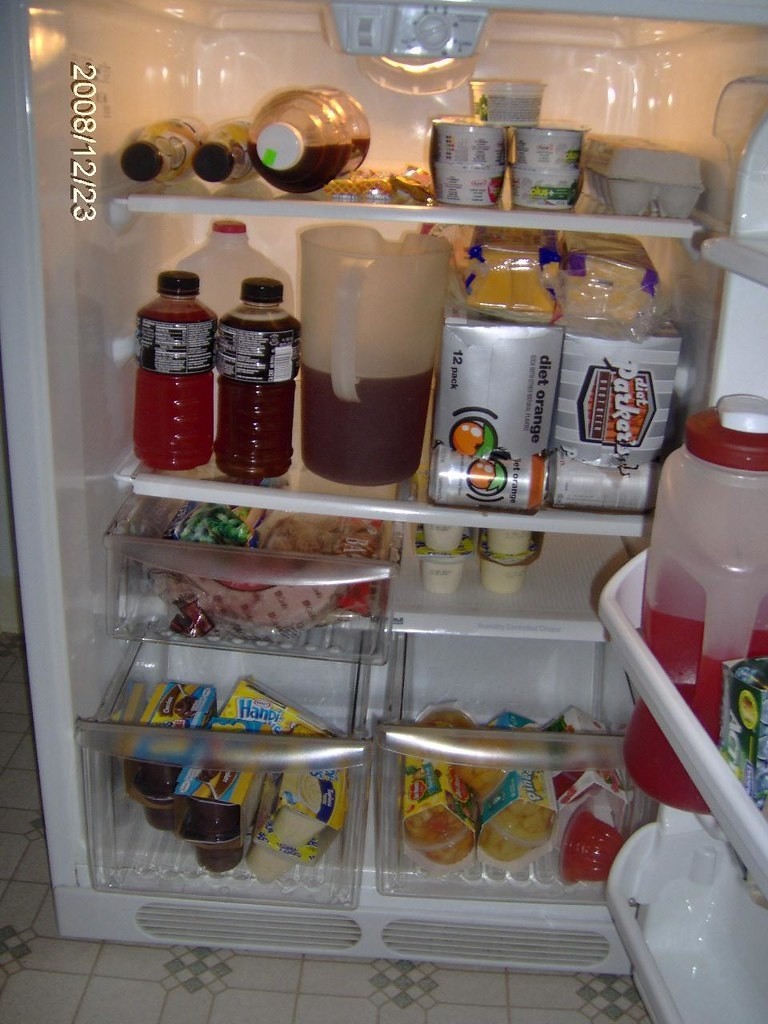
[
  {"xmin": 217, "ymin": 280, "xmax": 302, "ymax": 480},
  {"xmin": 624, "ymin": 393, "xmax": 767, "ymax": 812},
  {"xmin": 250, "ymin": 89, "xmax": 369, "ymax": 195},
  {"xmin": 174, "ymin": 219, "xmax": 291, "ymax": 443},
  {"xmin": 132, "ymin": 269, "xmax": 217, "ymax": 471},
  {"xmin": 120, "ymin": 114, "xmax": 197, "ymax": 184},
  {"xmin": 190, "ymin": 119, "xmax": 259, "ymax": 183}
]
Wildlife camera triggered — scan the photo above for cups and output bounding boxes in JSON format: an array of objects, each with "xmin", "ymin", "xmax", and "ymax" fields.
[
  {"xmin": 422, "ymin": 526, "xmax": 531, "ymax": 593},
  {"xmin": 404, "ymin": 706, "xmax": 624, "ymax": 884},
  {"xmin": 136, "ymin": 762, "xmax": 330, "ymax": 882}
]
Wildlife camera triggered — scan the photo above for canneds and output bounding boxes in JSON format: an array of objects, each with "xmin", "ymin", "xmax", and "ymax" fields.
[{"xmin": 428, "ymin": 443, "xmax": 663, "ymax": 516}]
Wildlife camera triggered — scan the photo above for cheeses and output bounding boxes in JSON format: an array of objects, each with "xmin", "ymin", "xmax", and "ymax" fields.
[{"xmin": 466, "ymin": 227, "xmax": 659, "ymax": 326}]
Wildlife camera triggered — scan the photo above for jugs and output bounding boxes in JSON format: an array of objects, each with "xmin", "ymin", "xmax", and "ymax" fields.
[{"xmin": 295, "ymin": 224, "xmax": 454, "ymax": 485}]
